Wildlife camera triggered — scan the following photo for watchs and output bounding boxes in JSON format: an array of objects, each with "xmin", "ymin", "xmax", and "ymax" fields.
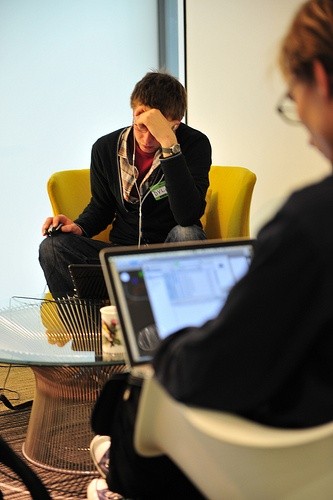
[{"xmin": 159, "ymin": 143, "xmax": 182, "ymax": 154}]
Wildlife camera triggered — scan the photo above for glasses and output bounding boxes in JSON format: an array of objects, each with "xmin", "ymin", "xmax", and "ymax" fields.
[{"xmin": 275, "ymin": 79, "xmax": 308, "ymax": 125}]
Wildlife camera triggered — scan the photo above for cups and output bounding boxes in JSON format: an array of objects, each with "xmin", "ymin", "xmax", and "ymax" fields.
[{"xmin": 99, "ymin": 305, "xmax": 127, "ymax": 353}]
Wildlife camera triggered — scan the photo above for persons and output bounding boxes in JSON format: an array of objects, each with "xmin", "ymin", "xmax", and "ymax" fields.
[
  {"xmin": 37, "ymin": 70, "xmax": 214, "ymax": 298},
  {"xmin": 84, "ymin": 1, "xmax": 333, "ymax": 499}
]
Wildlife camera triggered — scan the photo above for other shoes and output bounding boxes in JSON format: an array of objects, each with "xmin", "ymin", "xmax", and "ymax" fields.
[
  {"xmin": 87, "ymin": 478, "xmax": 124, "ymax": 500},
  {"xmin": 89, "ymin": 435, "xmax": 110, "ymax": 478}
]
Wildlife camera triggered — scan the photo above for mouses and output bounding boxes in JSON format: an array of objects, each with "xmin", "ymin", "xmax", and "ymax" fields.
[{"xmin": 45, "ymin": 222, "xmax": 63, "ymax": 237}]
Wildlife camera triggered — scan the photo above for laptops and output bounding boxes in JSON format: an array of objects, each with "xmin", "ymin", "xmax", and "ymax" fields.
[
  {"xmin": 69, "ymin": 264, "xmax": 109, "ymax": 301},
  {"xmin": 100, "ymin": 236, "xmax": 255, "ymax": 379}
]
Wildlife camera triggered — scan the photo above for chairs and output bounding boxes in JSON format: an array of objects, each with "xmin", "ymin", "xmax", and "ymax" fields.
[{"xmin": 132, "ymin": 374, "xmax": 333, "ymax": 500}]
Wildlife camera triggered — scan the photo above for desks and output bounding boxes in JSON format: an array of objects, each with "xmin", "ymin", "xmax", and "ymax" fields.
[{"xmin": 0, "ymin": 299, "xmax": 155, "ymax": 476}]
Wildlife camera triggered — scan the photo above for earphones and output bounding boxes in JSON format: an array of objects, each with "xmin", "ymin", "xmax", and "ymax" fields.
[{"xmin": 172, "ymin": 125, "xmax": 178, "ymax": 131}]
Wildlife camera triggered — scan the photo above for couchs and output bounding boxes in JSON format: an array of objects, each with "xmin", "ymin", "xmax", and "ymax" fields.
[{"xmin": 40, "ymin": 164, "xmax": 256, "ymax": 345}]
